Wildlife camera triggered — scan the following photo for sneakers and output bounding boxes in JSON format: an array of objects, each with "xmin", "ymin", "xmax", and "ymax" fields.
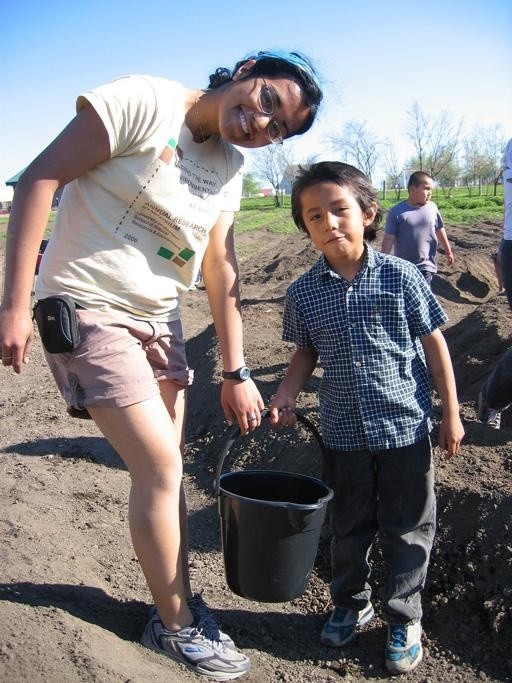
[
  {"xmin": 140, "ymin": 594, "xmax": 251, "ymax": 682},
  {"xmin": 384, "ymin": 623, "xmax": 422, "ymax": 675},
  {"xmin": 321, "ymin": 601, "xmax": 374, "ymax": 649}
]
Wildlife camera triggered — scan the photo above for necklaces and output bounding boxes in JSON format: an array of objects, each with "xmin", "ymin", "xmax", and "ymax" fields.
[{"xmin": 193, "ymin": 93, "xmax": 219, "ymax": 146}]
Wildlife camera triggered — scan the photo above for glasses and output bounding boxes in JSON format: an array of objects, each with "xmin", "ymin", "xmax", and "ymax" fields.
[{"xmin": 260, "ymin": 77, "xmax": 284, "ymax": 145}]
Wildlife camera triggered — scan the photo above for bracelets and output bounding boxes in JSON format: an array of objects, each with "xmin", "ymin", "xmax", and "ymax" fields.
[{"xmin": 446, "ymin": 251, "xmax": 454, "ymax": 254}]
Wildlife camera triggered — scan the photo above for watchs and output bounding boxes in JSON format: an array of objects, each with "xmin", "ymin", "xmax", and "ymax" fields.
[{"xmin": 220, "ymin": 364, "xmax": 251, "ymax": 383}]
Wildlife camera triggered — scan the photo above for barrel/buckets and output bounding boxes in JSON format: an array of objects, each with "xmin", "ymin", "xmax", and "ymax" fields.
[{"xmin": 212, "ymin": 408, "xmax": 333, "ymax": 604}]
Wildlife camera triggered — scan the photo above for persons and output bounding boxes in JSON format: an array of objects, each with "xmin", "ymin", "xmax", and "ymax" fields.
[
  {"xmin": 380, "ymin": 170, "xmax": 454, "ymax": 285},
  {"xmin": 0, "ymin": 49, "xmax": 324, "ymax": 681},
  {"xmin": 477, "ymin": 136, "xmax": 512, "ymax": 431},
  {"xmin": 267, "ymin": 159, "xmax": 466, "ymax": 674}
]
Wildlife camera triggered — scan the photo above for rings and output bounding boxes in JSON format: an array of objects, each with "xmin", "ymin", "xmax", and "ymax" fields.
[
  {"xmin": 248, "ymin": 414, "xmax": 257, "ymax": 421},
  {"xmin": 1, "ymin": 355, "xmax": 12, "ymax": 360}
]
